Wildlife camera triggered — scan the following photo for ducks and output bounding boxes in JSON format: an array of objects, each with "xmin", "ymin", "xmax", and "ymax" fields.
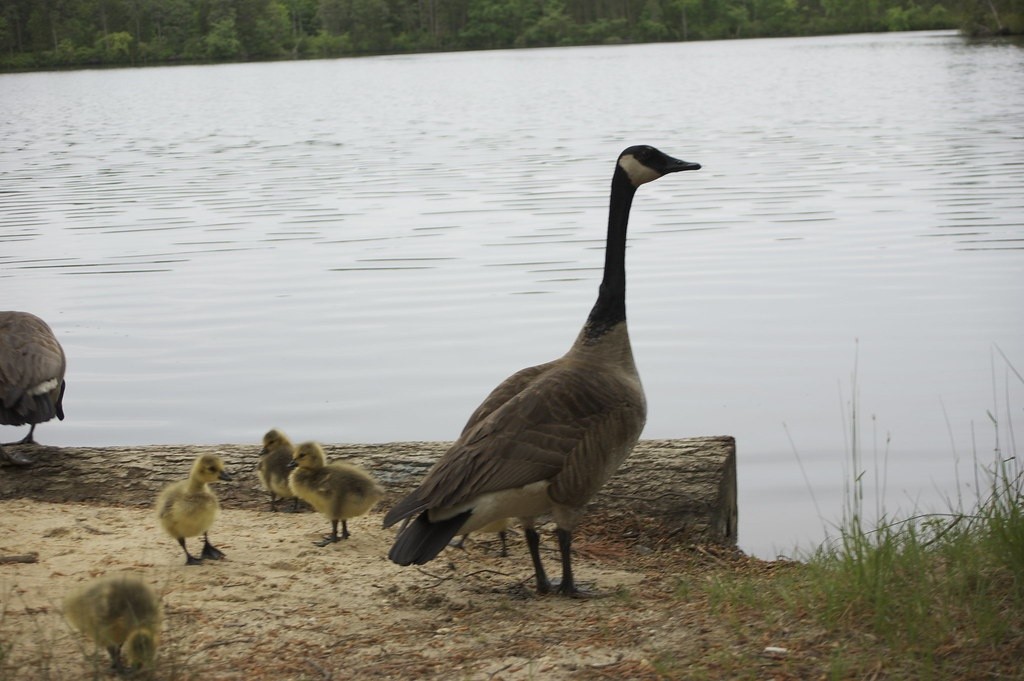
[
  {"xmin": 155, "ymin": 454, "xmax": 233, "ymax": 566},
  {"xmin": 64, "ymin": 577, "xmax": 160, "ymax": 674},
  {"xmin": 256, "ymin": 430, "xmax": 383, "ymax": 547}
]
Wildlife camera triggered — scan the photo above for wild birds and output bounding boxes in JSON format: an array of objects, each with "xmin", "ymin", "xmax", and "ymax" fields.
[
  {"xmin": 385, "ymin": 144, "xmax": 702, "ymax": 597},
  {"xmin": 0, "ymin": 312, "xmax": 67, "ymax": 442}
]
